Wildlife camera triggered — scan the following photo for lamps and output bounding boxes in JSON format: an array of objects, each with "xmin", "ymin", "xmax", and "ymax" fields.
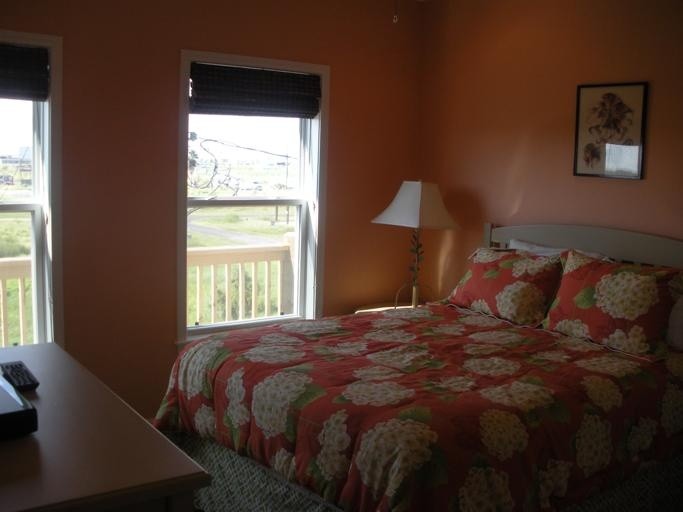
[{"xmin": 370, "ymin": 179, "xmax": 462, "ymax": 307}]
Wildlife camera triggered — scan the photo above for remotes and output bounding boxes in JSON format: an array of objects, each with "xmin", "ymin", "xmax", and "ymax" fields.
[{"xmin": 0, "ymin": 360, "xmax": 40, "ymax": 393}]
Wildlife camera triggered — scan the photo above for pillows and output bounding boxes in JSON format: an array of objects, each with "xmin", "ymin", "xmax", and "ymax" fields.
[{"xmin": 437, "ymin": 237, "xmax": 682, "ymax": 361}]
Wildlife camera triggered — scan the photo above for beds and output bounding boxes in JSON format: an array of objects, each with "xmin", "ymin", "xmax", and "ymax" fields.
[{"xmin": 153, "ymin": 217, "xmax": 683, "ymax": 512}]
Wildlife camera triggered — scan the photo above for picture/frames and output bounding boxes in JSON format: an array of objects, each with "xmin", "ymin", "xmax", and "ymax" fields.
[{"xmin": 572, "ymin": 81, "xmax": 649, "ymax": 180}]
